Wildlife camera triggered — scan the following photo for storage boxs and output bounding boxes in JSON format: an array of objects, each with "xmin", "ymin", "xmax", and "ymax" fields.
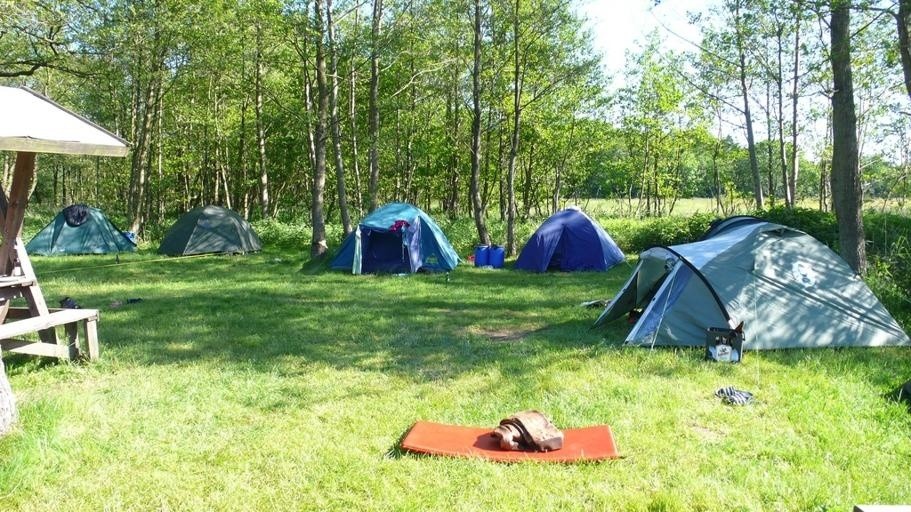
[{"xmin": 706, "ymin": 322, "xmax": 746, "ymax": 366}]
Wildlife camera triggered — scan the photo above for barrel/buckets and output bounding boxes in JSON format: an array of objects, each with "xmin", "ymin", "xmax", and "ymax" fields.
[
  {"xmin": 705, "ymin": 327, "xmax": 745, "ymax": 363},
  {"xmin": 474, "ymin": 246, "xmax": 489, "ymax": 267},
  {"xmin": 489, "ymin": 246, "xmax": 504, "ymax": 269}
]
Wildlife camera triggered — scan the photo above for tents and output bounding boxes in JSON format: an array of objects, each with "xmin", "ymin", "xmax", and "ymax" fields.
[
  {"xmin": 317, "ymin": 202, "xmax": 465, "ymax": 275},
  {"xmin": 21, "ymin": 203, "xmax": 143, "ymax": 257},
  {"xmin": 152, "ymin": 201, "xmax": 262, "ymax": 257},
  {"xmin": 590, "ymin": 219, "xmax": 911, "ymax": 353},
  {"xmin": 515, "ymin": 206, "xmax": 625, "ymax": 274},
  {"xmin": 700, "ymin": 216, "xmax": 759, "ymax": 239}
]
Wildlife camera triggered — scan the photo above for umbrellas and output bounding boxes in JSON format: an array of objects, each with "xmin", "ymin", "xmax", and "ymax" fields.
[{"xmin": 0, "ymin": 80, "xmax": 131, "ymax": 163}]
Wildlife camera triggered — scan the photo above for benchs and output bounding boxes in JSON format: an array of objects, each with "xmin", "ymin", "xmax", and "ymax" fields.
[{"xmin": 1, "ymin": 306, "xmax": 99, "ymax": 366}]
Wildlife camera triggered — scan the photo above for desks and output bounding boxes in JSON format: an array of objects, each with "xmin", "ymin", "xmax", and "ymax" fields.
[{"xmin": 1, "ymin": 267, "xmax": 38, "ymax": 319}]
